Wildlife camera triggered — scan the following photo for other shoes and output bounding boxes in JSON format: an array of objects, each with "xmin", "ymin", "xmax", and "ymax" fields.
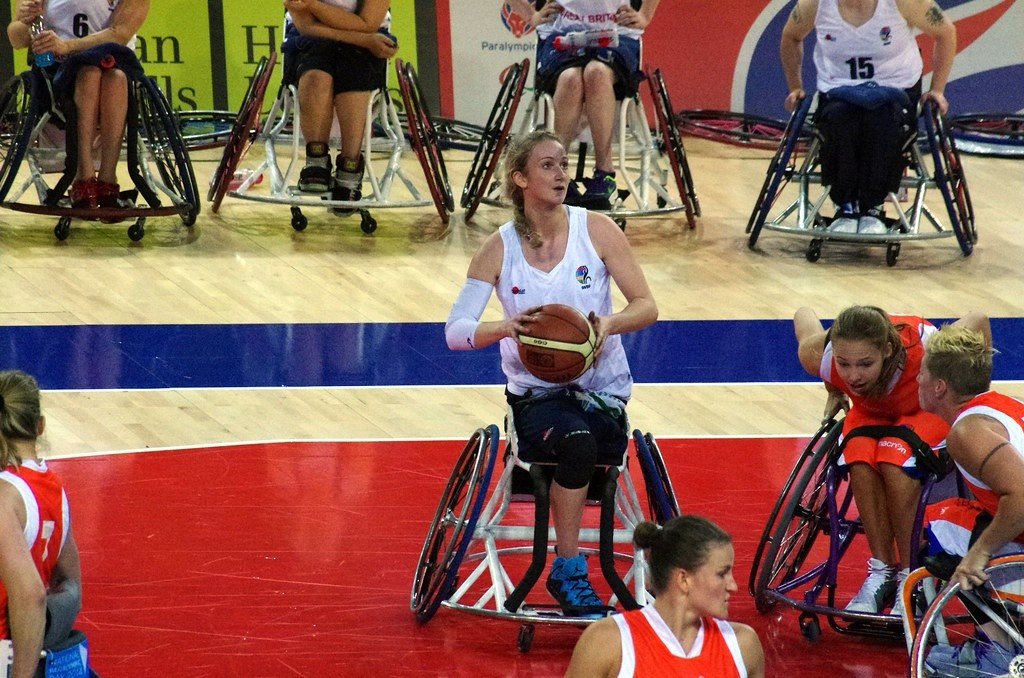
[
  {"xmin": 857, "ymin": 205, "xmax": 887, "ymax": 234},
  {"xmin": 826, "ymin": 205, "xmax": 857, "ymax": 234}
]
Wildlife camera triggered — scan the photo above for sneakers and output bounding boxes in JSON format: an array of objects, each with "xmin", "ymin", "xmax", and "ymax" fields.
[
  {"xmin": 890, "ymin": 568, "xmax": 909, "ymax": 615},
  {"xmin": 68, "ymin": 177, "xmax": 100, "ymax": 222},
  {"xmin": 545, "ymin": 545, "xmax": 607, "ymax": 618},
  {"xmin": 298, "ymin": 141, "xmax": 333, "ymax": 191},
  {"xmin": 332, "ymin": 153, "xmax": 365, "ymax": 218},
  {"xmin": 96, "ymin": 178, "xmax": 126, "ymax": 224},
  {"xmin": 925, "ymin": 629, "xmax": 1024, "ymax": 678},
  {"xmin": 585, "ymin": 167, "xmax": 618, "ymax": 206},
  {"xmin": 562, "ymin": 179, "xmax": 585, "ymax": 207},
  {"xmin": 841, "ymin": 558, "xmax": 898, "ymax": 621}
]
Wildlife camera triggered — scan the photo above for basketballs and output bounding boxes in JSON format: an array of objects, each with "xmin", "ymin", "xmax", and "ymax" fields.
[{"xmin": 517, "ymin": 303, "xmax": 597, "ymax": 383}]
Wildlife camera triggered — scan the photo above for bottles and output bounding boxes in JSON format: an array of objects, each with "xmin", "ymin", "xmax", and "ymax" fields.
[
  {"xmin": 28, "ymin": 10, "xmax": 55, "ymax": 68},
  {"xmin": 218, "ymin": 167, "xmax": 262, "ymax": 191},
  {"xmin": 552, "ymin": 26, "xmax": 620, "ymax": 52}
]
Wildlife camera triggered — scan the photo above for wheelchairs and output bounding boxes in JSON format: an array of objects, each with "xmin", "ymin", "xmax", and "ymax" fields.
[
  {"xmin": 745, "ymin": 101, "xmax": 978, "ymax": 268},
  {"xmin": 207, "ymin": 52, "xmax": 455, "ymax": 234},
  {"xmin": 409, "ymin": 405, "xmax": 684, "ymax": 651},
  {"xmin": 1, "ymin": 69, "xmax": 202, "ymax": 244},
  {"xmin": 747, "ymin": 401, "xmax": 1024, "ymax": 678},
  {"xmin": 460, "ymin": 58, "xmax": 701, "ymax": 232}
]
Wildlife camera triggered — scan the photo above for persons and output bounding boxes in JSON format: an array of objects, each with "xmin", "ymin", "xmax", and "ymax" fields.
[
  {"xmin": 283, "ymin": 0, "xmax": 398, "ymax": 218},
  {"xmin": 506, "ymin": 0, "xmax": 659, "ymax": 211},
  {"xmin": 0, "ymin": 370, "xmax": 83, "ymax": 678},
  {"xmin": 561, "ymin": 514, "xmax": 765, "ymax": 678},
  {"xmin": 445, "ymin": 130, "xmax": 658, "ymax": 629},
  {"xmin": 915, "ymin": 328, "xmax": 1024, "ymax": 678},
  {"xmin": 794, "ymin": 305, "xmax": 992, "ymax": 624},
  {"xmin": 7, "ymin": 0, "xmax": 150, "ymax": 224},
  {"xmin": 780, "ymin": 0, "xmax": 957, "ymax": 239}
]
